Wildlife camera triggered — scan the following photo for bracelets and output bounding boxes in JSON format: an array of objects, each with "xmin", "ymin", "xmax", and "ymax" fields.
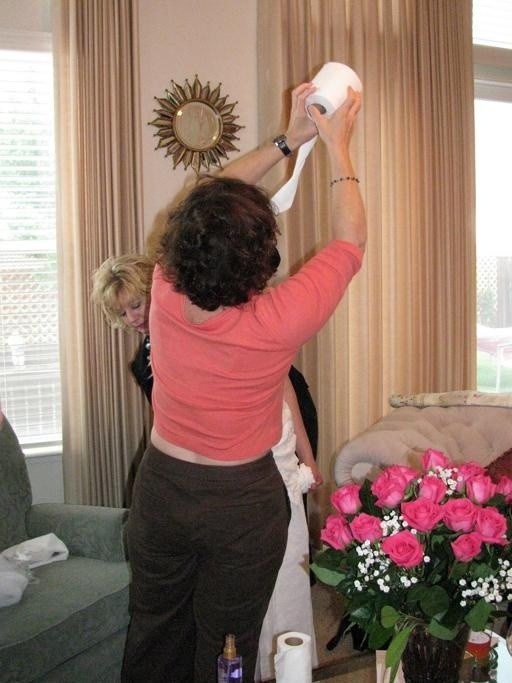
[
  {"xmin": 272, "ymin": 129, "xmax": 293, "ymax": 158},
  {"xmin": 326, "ymin": 172, "xmax": 363, "ymax": 183}
]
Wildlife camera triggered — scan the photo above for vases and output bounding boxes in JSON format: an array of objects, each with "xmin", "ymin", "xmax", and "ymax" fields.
[{"xmin": 399, "ymin": 618, "xmax": 470, "ymax": 682}]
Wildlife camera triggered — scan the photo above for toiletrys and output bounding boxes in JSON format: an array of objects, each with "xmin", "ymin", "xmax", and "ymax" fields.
[{"xmin": 217, "ymin": 628, "xmax": 245, "ymax": 683}]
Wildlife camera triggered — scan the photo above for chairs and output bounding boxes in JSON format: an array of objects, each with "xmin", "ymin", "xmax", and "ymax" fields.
[{"xmin": 0, "ymin": 412, "xmax": 135, "ymax": 682}]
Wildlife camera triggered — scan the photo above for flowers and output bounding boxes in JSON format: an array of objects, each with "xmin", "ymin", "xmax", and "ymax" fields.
[{"xmin": 306, "ymin": 449, "xmax": 512, "ymax": 683}]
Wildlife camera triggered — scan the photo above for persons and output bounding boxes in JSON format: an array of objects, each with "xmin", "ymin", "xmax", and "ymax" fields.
[
  {"xmin": 114, "ymin": 76, "xmax": 371, "ymax": 682},
  {"xmin": 257, "ymin": 372, "xmax": 324, "ymax": 681},
  {"xmin": 93, "ymin": 260, "xmax": 321, "ymax": 597}
]
